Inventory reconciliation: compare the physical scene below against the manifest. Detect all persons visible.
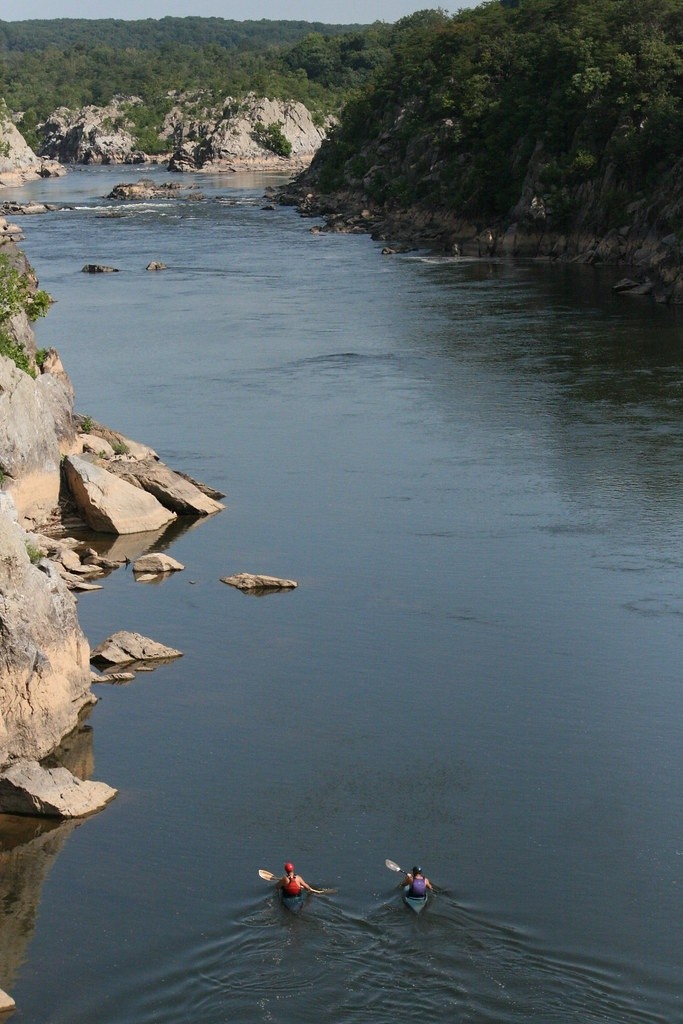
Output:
[401,865,433,898]
[275,863,319,898]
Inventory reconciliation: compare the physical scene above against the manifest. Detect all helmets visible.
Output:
[412,866,423,875]
[285,863,295,871]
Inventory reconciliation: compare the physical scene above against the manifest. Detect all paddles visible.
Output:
[385,858,439,893]
[258,869,338,894]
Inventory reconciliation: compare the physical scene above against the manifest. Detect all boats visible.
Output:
[282,897,304,918]
[403,875,428,914]
